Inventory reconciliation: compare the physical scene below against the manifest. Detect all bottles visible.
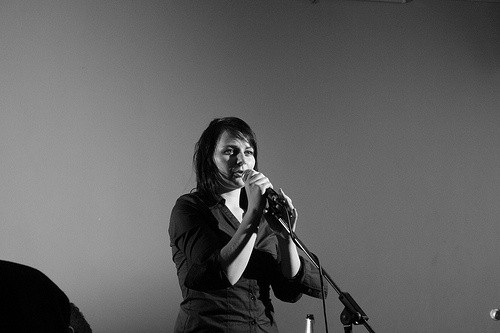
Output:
[304,314,315,333]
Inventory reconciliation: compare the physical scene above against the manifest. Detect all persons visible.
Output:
[168,116,327,333]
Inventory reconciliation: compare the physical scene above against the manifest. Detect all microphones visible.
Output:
[490,308,500,320]
[242,169,285,207]
